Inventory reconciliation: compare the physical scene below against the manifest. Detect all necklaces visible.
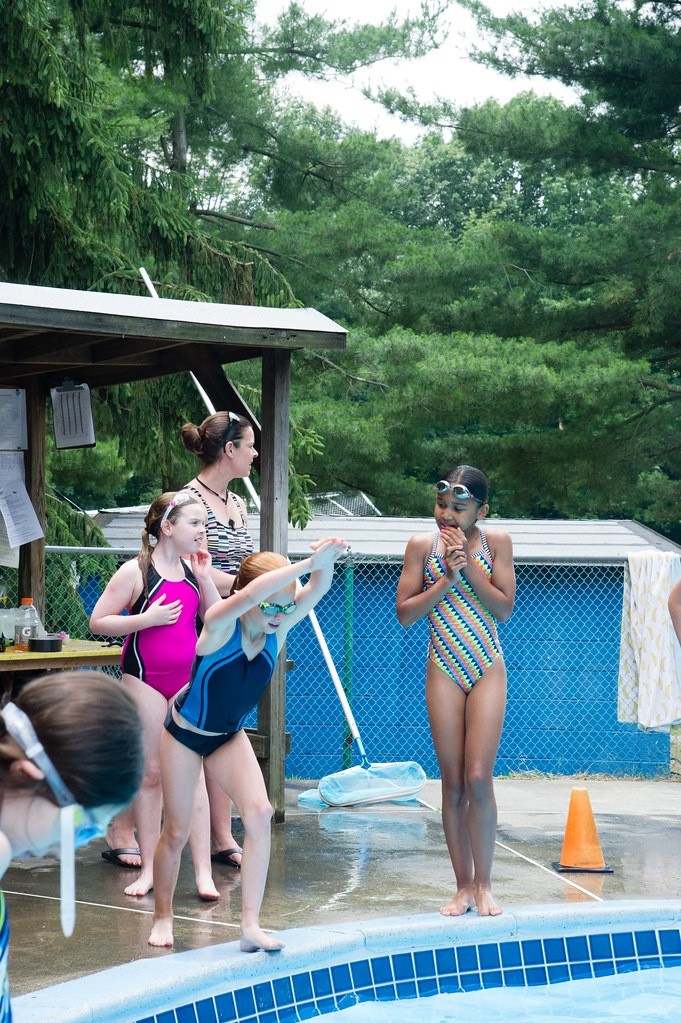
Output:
[195,475,235,532]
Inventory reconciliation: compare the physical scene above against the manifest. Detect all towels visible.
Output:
[617,549,681,733]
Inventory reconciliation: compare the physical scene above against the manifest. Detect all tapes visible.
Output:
[28,637,63,652]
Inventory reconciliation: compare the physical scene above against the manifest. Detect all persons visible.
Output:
[147,539,351,953]
[396,466,516,917]
[89,489,222,900]
[0,668,147,1022]
[102,410,259,870]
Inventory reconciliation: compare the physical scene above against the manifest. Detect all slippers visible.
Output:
[212,848,243,867]
[101,848,142,869]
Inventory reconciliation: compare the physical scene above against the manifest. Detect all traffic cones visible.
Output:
[550,788,614,873]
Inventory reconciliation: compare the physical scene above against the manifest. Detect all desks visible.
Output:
[0,638,123,699]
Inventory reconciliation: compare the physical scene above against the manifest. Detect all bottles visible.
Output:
[14,598,38,651]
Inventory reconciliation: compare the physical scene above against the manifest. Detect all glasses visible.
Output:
[222,411,240,453]
[435,480,485,506]
[160,488,201,529]
[233,589,297,617]
[33,760,104,851]
